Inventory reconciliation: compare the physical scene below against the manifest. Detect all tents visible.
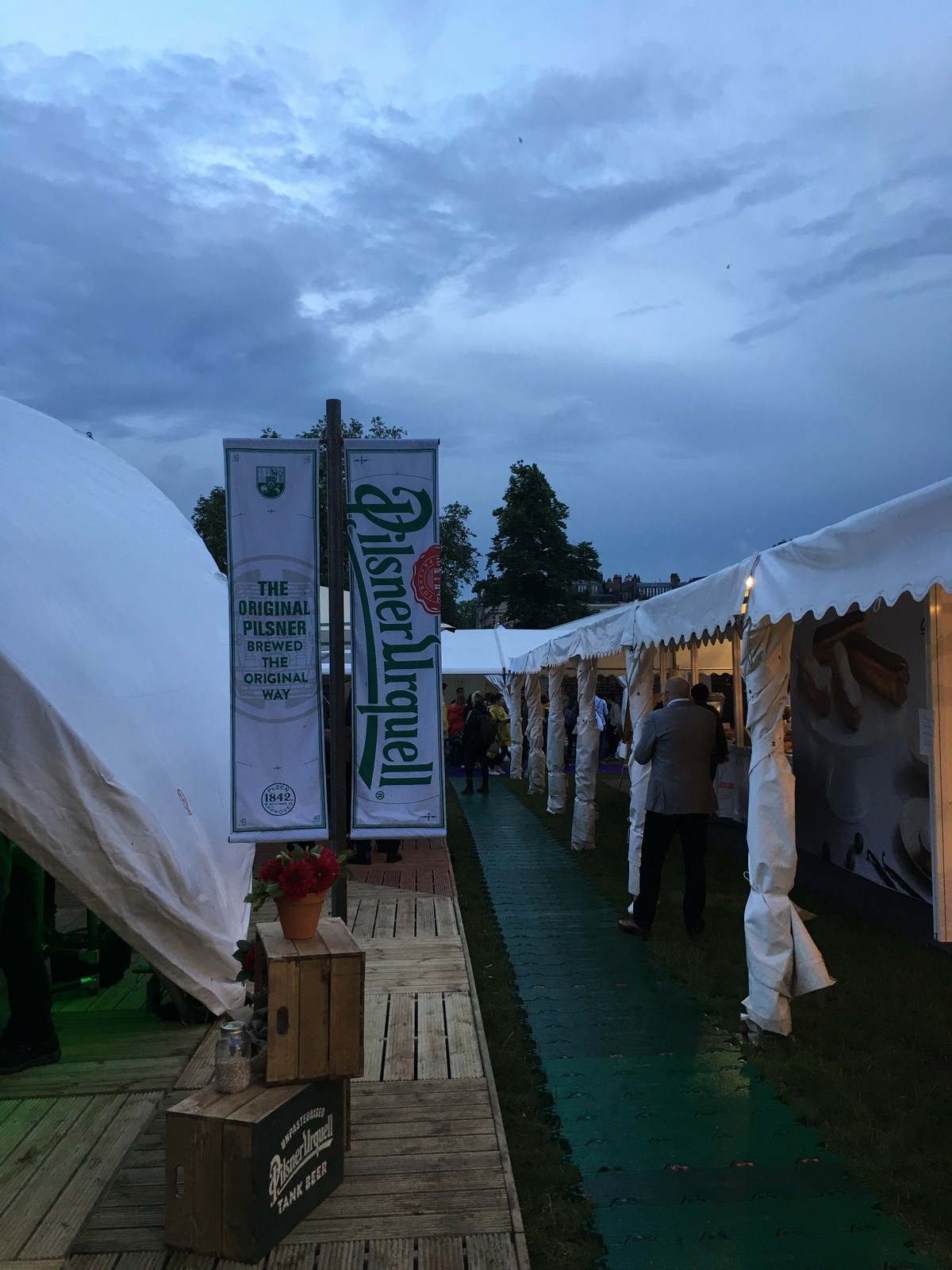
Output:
[504,478,952,1037]
[0,395,257,1017]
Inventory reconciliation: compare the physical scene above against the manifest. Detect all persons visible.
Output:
[690,683,729,784]
[541,694,549,754]
[594,695,609,765]
[344,687,403,865]
[720,675,747,727]
[0,833,61,1074]
[616,676,718,937]
[489,693,510,774]
[606,693,621,760]
[442,683,473,769]
[563,697,578,758]
[460,691,491,795]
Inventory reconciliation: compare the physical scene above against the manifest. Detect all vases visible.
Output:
[275,890,328,939]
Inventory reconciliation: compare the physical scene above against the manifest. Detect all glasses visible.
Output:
[659,690,669,700]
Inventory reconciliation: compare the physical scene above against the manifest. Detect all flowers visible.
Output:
[242,842,353,912]
[233,936,268,1014]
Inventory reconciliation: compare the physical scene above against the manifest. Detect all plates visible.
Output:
[900,797,932,882]
[800,660,907,745]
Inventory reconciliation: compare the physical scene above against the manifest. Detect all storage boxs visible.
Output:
[255,917,366,1086]
[163,1076,352,1265]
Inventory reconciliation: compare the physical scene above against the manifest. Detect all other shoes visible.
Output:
[690,920,707,937]
[461,787,474,795]
[488,767,501,775]
[494,764,506,774]
[477,786,489,794]
[619,920,650,939]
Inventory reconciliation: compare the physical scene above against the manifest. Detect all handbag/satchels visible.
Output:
[449,731,463,747]
[572,725,577,734]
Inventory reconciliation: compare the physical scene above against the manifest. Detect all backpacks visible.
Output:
[595,699,604,732]
[474,708,498,742]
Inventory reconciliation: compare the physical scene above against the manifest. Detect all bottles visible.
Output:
[214,1021,250,1093]
